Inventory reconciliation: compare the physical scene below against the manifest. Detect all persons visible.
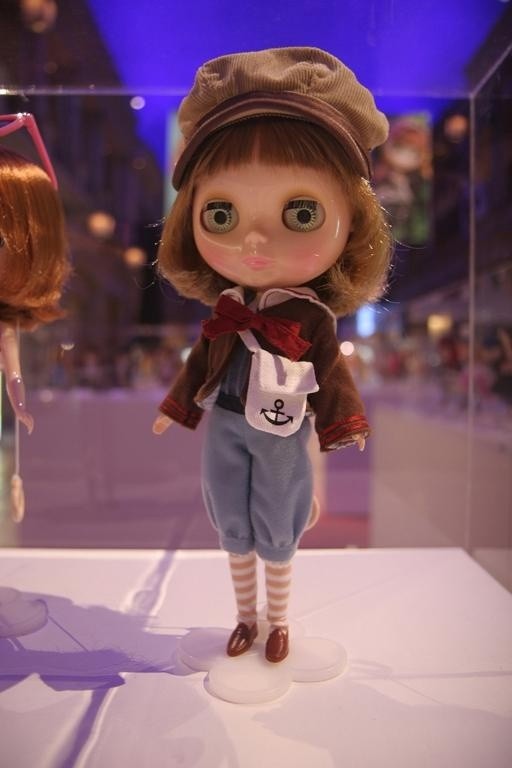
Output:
[151,47,391,664]
